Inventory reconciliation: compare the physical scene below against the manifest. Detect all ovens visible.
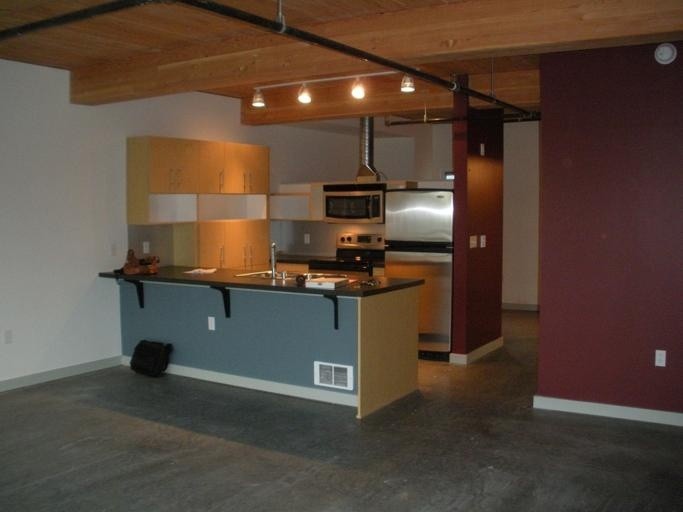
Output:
[310,267,370,277]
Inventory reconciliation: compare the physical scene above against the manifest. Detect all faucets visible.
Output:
[270,243,278,278]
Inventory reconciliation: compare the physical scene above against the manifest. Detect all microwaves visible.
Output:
[322,192,384,225]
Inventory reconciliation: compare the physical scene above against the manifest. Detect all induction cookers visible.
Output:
[310,250,386,266]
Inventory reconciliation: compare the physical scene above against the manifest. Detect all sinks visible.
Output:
[284,272,349,283]
[233,269,284,279]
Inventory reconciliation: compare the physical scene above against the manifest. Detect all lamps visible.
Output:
[249,69,416,109]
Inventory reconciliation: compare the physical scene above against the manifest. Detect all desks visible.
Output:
[96,266,426,420]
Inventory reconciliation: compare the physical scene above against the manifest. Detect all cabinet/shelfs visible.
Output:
[124,136,198,194]
[125,195,268,224]
[223,221,272,269]
[173,222,223,266]
[198,139,223,194]
[223,141,268,194]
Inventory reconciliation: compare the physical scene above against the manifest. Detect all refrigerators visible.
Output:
[382,190,454,357]
